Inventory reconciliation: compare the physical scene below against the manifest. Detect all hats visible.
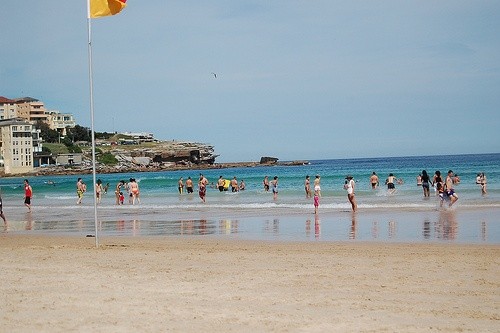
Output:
[345,175,353,179]
[97,178,102,183]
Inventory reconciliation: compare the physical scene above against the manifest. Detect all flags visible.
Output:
[87,0,127,20]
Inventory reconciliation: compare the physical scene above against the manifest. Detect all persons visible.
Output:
[385,171,406,193]
[304,173,322,214]
[369,171,379,190]
[216,175,245,192]
[96,178,109,203]
[416,170,461,208]
[0,192,6,224]
[76,177,87,205]
[197,174,209,205]
[476,172,488,195]
[343,174,359,213]
[264,175,280,200]
[115,178,142,205]
[178,176,194,194]
[23,179,33,213]
